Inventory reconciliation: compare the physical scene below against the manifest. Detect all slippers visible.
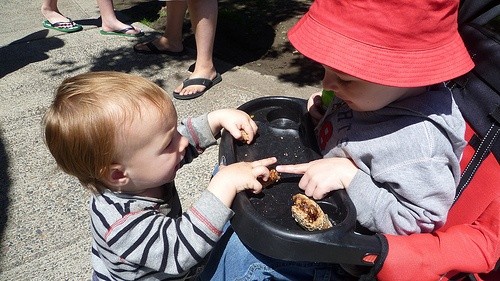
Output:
[100,24,145,37]
[172,72,222,100]
[134,38,184,56]
[43,16,82,33]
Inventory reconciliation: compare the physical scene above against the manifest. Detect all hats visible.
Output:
[286,0,476,88]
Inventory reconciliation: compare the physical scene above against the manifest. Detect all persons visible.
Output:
[132,0,222,100]
[40,0,145,36]
[41,71,277,281]
[204,0,475,281]
